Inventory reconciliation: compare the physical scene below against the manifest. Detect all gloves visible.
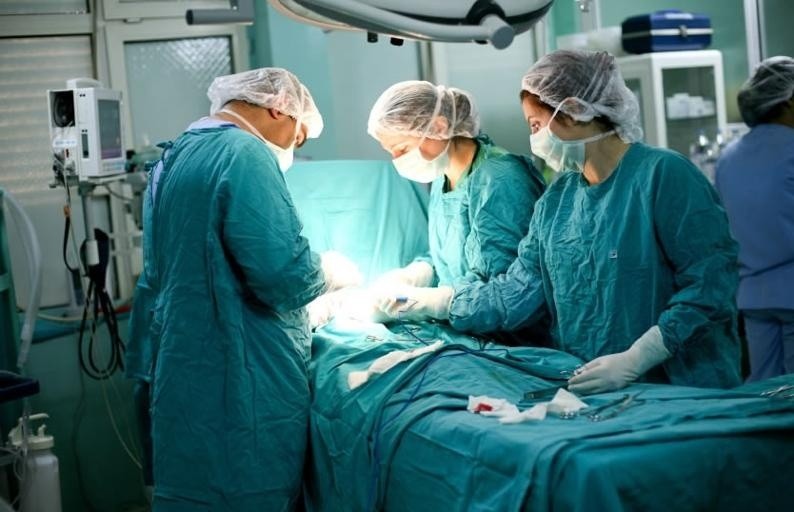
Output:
[321,251,362,291]
[566,322,672,398]
[374,287,455,322]
[384,262,435,287]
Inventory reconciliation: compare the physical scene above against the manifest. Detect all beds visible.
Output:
[246,160,794,511]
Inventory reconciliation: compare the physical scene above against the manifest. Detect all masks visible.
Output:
[529,97,596,176]
[392,117,456,184]
[259,108,301,176]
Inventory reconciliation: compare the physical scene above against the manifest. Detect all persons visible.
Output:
[353,77,553,346]
[371,46,745,405]
[700,56,794,379]
[122,65,335,509]
